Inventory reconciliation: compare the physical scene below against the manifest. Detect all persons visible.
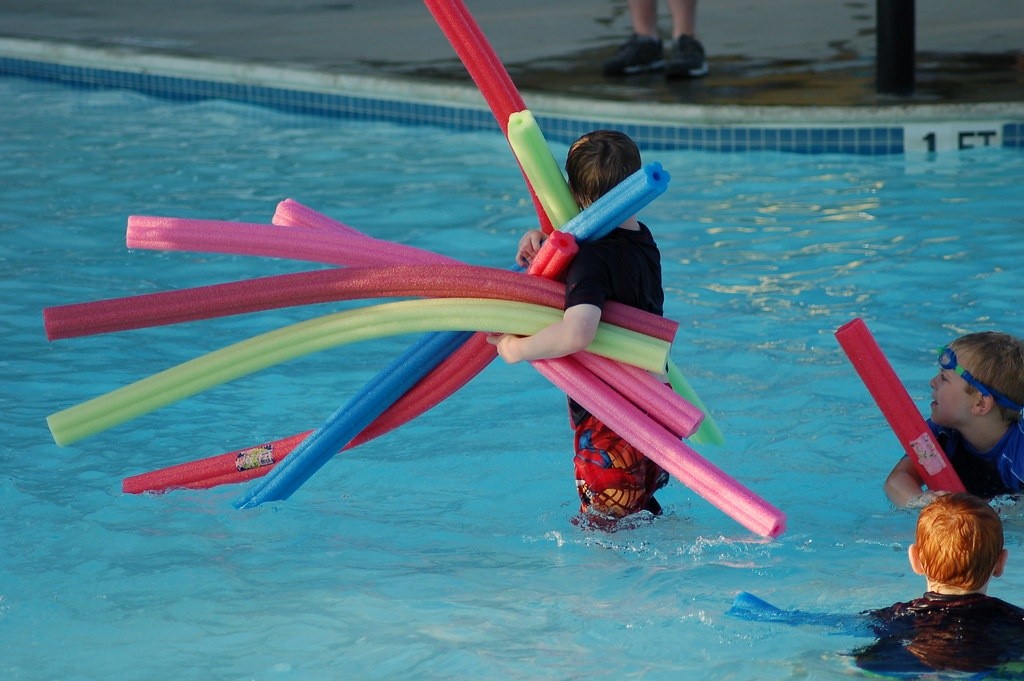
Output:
[598,0,709,79]
[855,490,1024,681]
[883,331,1024,512]
[486,128,681,535]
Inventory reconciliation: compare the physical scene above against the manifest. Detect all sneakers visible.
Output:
[665,33,708,78]
[602,33,665,75]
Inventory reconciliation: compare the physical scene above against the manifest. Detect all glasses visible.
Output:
[939,341,1024,412]
